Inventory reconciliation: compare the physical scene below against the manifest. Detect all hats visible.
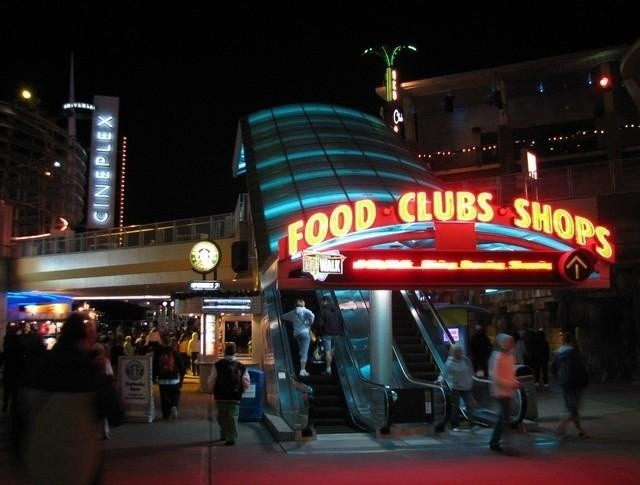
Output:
[222,341,238,353]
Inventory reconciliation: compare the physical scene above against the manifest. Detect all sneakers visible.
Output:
[489,441,506,452]
[298,367,312,376]
[226,440,236,446]
[169,406,177,422]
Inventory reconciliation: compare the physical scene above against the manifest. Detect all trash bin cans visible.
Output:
[239,368,264,424]
[195,357,222,393]
[512,364,539,419]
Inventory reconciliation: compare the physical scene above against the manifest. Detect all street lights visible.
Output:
[360,40,420,134]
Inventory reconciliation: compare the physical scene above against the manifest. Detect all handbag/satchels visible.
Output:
[312,345,326,361]
[309,329,317,343]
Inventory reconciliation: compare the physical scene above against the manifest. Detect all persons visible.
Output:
[550,332,594,440]
[313,295,343,377]
[469,315,552,388]
[1,306,253,484]
[485,333,528,453]
[432,344,481,434]
[281,298,317,377]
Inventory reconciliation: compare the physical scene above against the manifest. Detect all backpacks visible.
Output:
[158,349,178,374]
